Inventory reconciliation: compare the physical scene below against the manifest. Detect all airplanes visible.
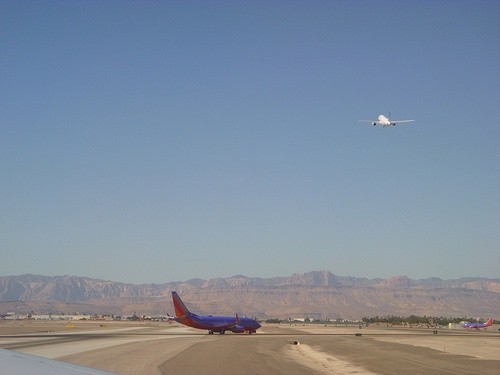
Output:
[168,292,261,336]
[464,316,494,331]
[360,113,416,127]
